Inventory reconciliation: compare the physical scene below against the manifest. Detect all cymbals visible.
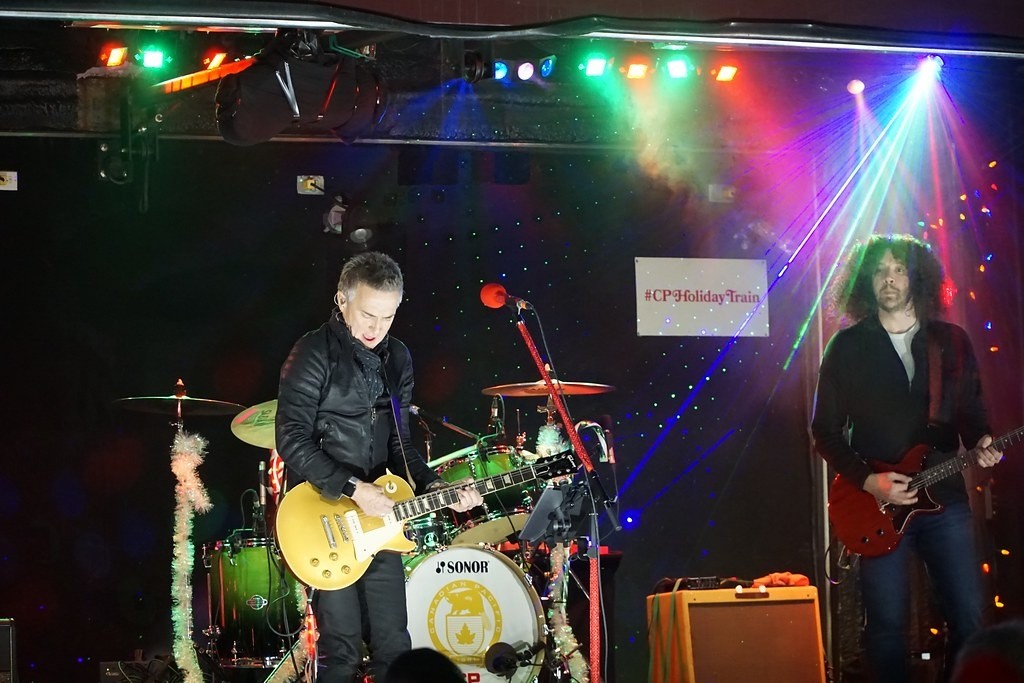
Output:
[480,362,615,397]
[230,398,278,450]
[114,376,248,417]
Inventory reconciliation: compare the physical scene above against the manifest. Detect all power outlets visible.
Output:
[0,171,18,191]
[708,183,738,203]
[297,175,325,195]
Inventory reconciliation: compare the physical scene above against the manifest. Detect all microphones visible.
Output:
[647,585,827,683]
[487,398,498,435]
[480,283,532,311]
[537,364,551,414]
[494,657,517,673]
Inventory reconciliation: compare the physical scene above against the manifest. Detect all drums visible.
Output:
[433,443,534,549]
[360,542,549,683]
[206,537,301,669]
[403,516,446,556]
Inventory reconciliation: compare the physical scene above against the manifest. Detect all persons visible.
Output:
[384,647,468,683]
[809,234,1003,683]
[274,252,483,683]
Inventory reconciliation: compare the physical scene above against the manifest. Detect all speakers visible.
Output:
[0,625,15,683]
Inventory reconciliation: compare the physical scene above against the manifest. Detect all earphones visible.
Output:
[340,298,345,306]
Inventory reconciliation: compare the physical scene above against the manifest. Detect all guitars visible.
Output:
[827,424,1024,559]
[271,448,579,593]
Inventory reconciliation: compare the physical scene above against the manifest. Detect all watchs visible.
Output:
[342,476,359,498]
